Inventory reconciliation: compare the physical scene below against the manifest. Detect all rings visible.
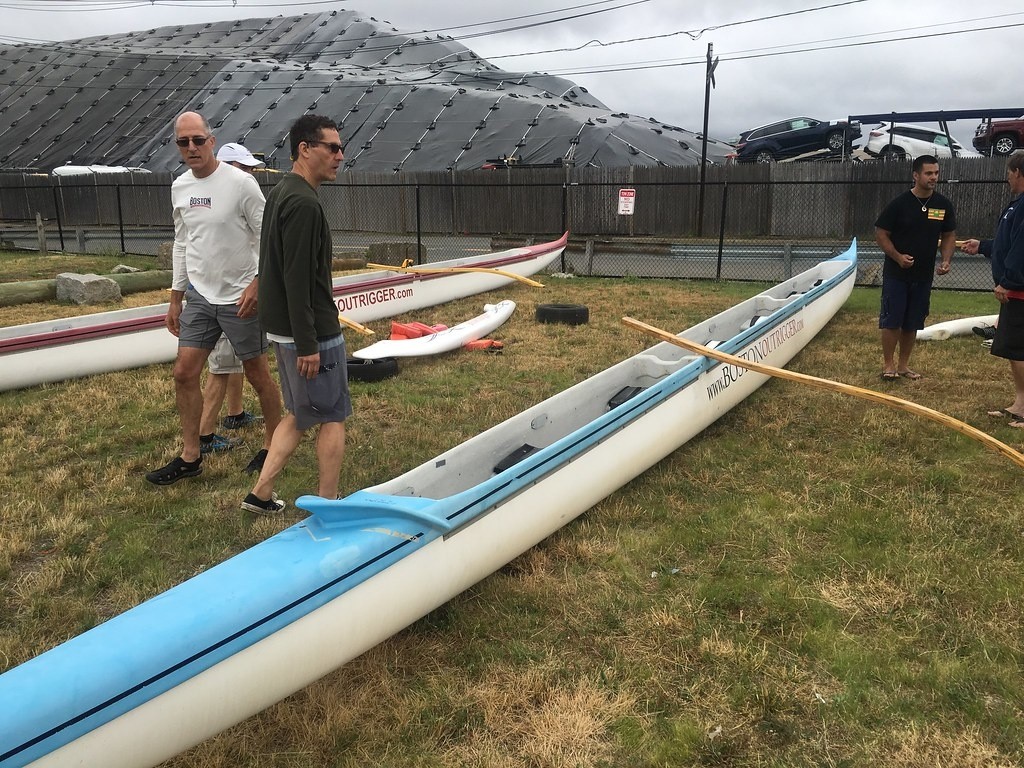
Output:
[252,307,256,311]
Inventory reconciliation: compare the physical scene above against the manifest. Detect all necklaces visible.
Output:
[912,189,932,212]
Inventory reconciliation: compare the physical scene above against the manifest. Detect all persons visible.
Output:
[874,155,958,380]
[241,115,353,515]
[144,110,283,484]
[961,148,1024,428]
[199,142,267,453]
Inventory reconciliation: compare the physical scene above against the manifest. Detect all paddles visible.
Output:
[365,262,546,289]
[165,287,376,336]
[621,315,1024,471]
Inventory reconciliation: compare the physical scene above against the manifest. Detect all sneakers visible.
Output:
[336,493,343,500]
[240,492,285,516]
[972,323,997,339]
[221,412,265,430]
[199,435,244,454]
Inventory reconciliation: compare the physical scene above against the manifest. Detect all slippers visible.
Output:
[897,370,923,380]
[880,371,901,381]
[1008,417,1024,429]
[987,409,1022,419]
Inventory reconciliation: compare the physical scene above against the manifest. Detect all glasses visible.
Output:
[175,134,211,148]
[298,139,345,155]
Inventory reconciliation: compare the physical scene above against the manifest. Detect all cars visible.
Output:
[863,121,985,169]
[972,114,1024,157]
[733,116,862,166]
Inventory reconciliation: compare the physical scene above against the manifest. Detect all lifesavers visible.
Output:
[347,356,400,384]
[536,303,591,327]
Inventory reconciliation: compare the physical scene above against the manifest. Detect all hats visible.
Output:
[216,143,266,169]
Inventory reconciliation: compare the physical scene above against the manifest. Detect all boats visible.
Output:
[352,298,516,360]
[0,229,569,396]
[1,236,859,768]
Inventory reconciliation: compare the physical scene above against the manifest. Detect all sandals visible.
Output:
[146,454,203,485]
[241,448,268,477]
